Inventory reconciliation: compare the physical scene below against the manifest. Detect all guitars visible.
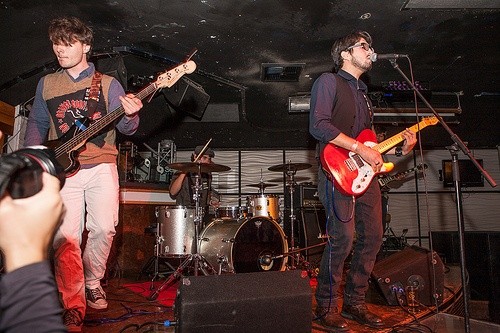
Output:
[38,47,199,178]
[320,116,442,197]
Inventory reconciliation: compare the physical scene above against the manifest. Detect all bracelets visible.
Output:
[395,148,402,157]
[352,141,358,151]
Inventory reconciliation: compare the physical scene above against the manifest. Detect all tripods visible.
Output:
[134,167,217,302]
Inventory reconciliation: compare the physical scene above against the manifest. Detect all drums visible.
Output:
[154,193,288,273]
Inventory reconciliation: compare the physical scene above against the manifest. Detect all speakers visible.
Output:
[367,243,445,307]
[282,208,327,271]
[172,270,313,333]
[161,76,210,120]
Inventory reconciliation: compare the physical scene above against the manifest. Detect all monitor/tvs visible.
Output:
[442,159,484,187]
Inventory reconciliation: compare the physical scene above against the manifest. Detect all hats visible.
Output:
[195,146,214,158]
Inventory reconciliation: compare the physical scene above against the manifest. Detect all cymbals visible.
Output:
[268,163,313,172]
[246,184,278,188]
[168,162,231,175]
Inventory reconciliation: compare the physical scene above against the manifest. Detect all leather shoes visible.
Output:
[340,303,385,329]
[316,305,351,330]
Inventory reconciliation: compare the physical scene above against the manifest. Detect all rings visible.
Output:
[376,162,379,165]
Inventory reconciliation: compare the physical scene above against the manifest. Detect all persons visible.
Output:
[169,146,222,213]
[0,172,69,333]
[309,31,417,330]
[23,16,143,331]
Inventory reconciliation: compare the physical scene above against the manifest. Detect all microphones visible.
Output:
[370,52,408,63]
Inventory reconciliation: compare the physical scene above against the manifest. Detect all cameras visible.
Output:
[0,143,66,204]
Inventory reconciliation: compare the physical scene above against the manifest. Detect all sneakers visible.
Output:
[62,308,84,332]
[85,286,108,309]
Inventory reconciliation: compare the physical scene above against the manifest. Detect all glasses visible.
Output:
[345,41,374,53]
